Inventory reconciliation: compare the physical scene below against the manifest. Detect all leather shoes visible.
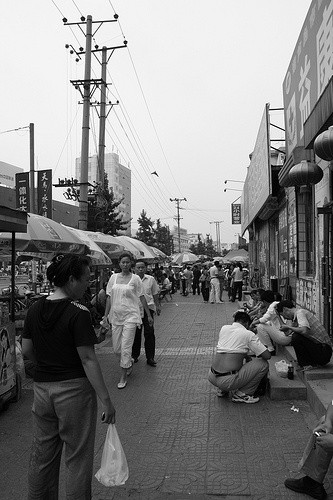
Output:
[132,357,138,363]
[147,357,158,364]
[285,476,328,500]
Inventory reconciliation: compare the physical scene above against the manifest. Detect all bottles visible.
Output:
[288,360,294,380]
[100,321,110,329]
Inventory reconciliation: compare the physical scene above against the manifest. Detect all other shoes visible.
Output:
[181,293,242,304]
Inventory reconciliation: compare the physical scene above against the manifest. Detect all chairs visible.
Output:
[157,282,173,302]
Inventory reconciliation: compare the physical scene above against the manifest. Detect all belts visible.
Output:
[211,366,237,377]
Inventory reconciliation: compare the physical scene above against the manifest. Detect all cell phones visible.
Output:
[102,412,105,421]
[315,432,320,438]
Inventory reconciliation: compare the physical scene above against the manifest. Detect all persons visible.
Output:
[159,261,244,304]
[101,253,154,388]
[243,288,282,325]
[250,290,295,356]
[130,259,161,366]
[78,279,108,329]
[208,312,271,404]
[275,301,333,372]
[22,253,117,500]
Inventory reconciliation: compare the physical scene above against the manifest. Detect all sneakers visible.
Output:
[296,365,313,372]
[117,380,128,389]
[232,392,260,404]
[217,389,229,397]
[126,358,134,376]
